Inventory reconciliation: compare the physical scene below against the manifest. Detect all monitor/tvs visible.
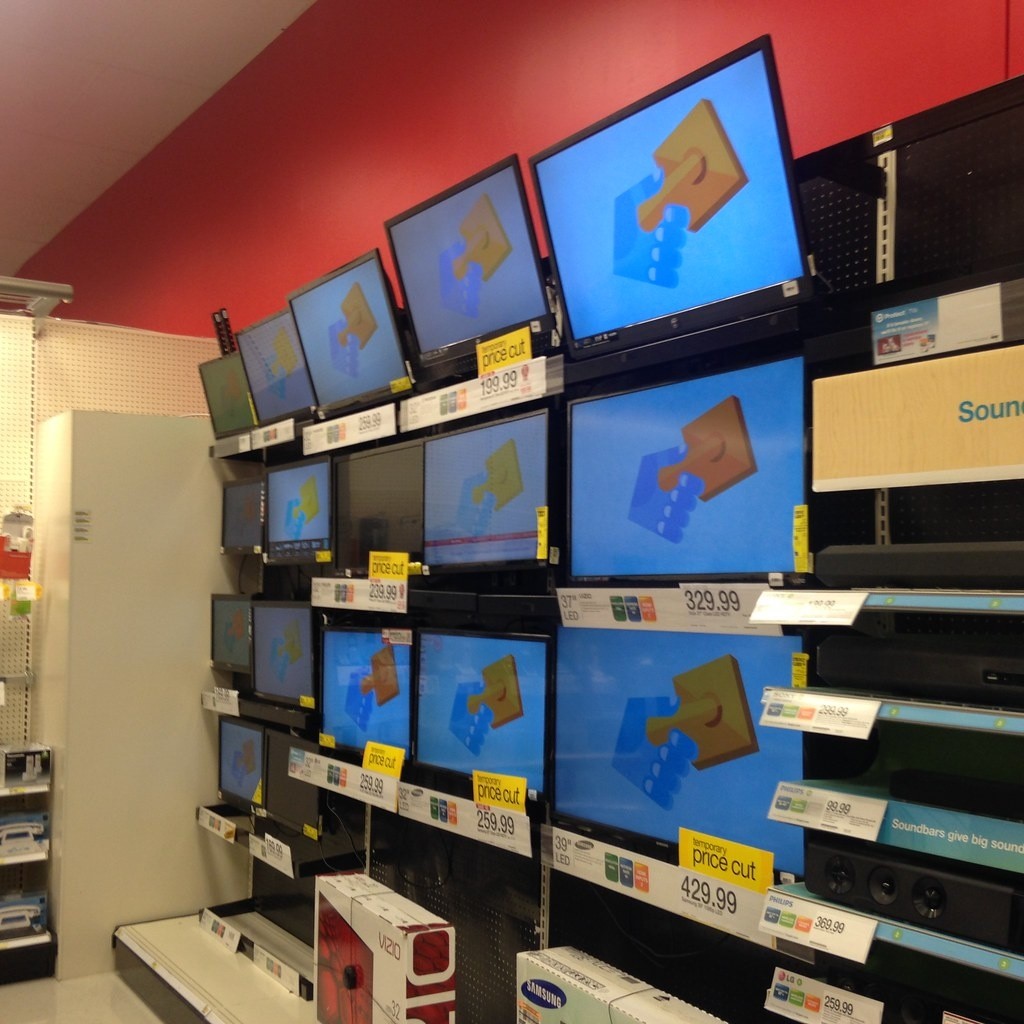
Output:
[200,36,817,891]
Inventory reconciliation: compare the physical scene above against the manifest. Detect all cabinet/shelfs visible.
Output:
[194,306,1024,1024]
[0,744,58,985]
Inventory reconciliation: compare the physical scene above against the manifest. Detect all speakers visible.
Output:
[803,837,1024,956]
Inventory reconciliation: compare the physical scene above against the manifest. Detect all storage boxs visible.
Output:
[513,948,730,1024]
[314,873,458,1024]
